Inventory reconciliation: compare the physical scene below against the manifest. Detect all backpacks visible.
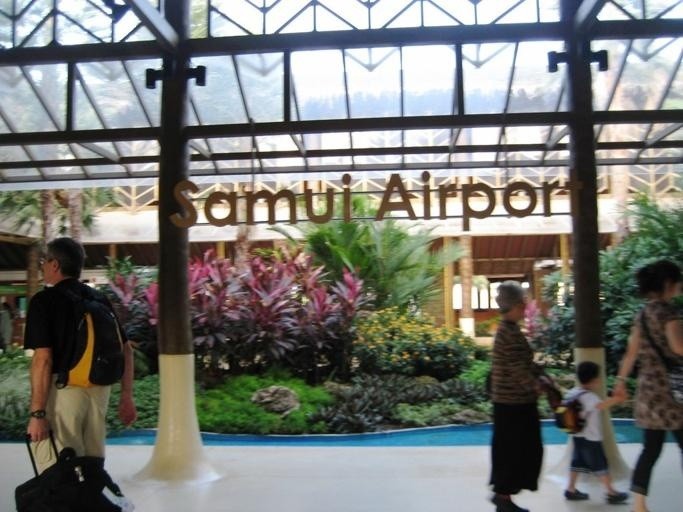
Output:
[46,286,124,390]
[554,391,592,436]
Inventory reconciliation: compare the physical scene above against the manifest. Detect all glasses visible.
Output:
[39,256,49,264]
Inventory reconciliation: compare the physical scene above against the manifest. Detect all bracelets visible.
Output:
[29,409,48,420]
[615,376,627,383]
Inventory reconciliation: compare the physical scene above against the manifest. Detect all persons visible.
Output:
[562,361,629,505]
[485,281,557,512]
[610,258,682,512]
[23,237,138,476]
[0,302,16,354]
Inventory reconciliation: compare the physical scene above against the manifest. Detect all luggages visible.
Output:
[15,429,124,512]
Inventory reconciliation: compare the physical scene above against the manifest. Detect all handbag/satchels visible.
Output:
[486,371,490,394]
[663,358,682,374]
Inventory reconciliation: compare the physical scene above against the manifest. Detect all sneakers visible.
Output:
[564,489,589,500]
[607,492,628,503]
[492,494,529,512]
